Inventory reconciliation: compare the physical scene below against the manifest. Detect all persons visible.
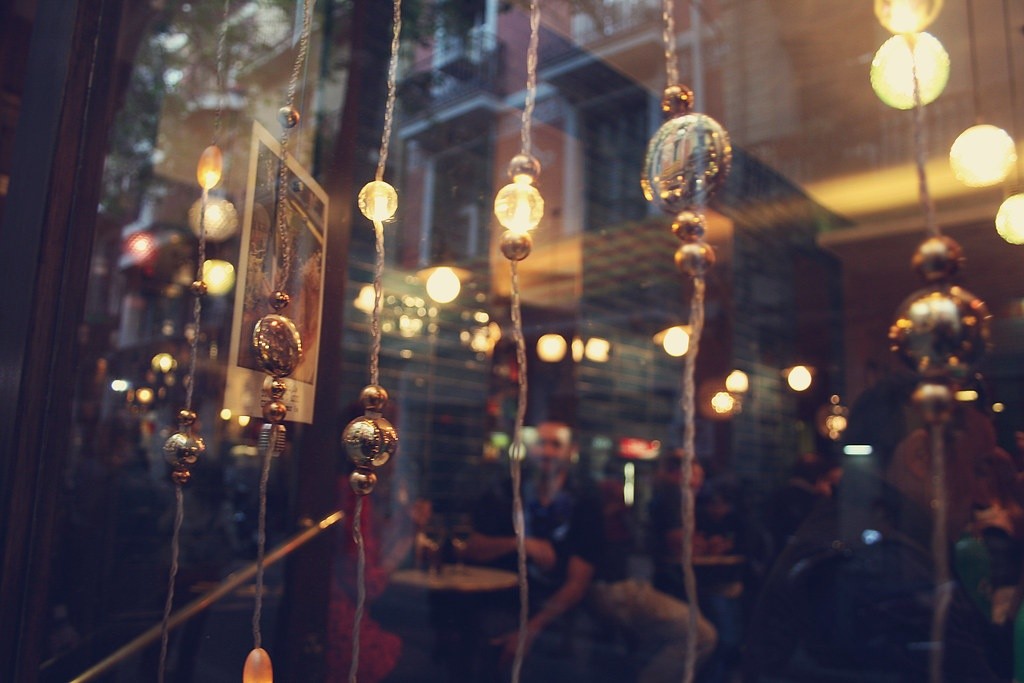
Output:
[585,376,1022,683]
[446,416,602,676]
[327,397,433,683]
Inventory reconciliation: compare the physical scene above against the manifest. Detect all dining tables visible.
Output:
[390,560,524,682]
